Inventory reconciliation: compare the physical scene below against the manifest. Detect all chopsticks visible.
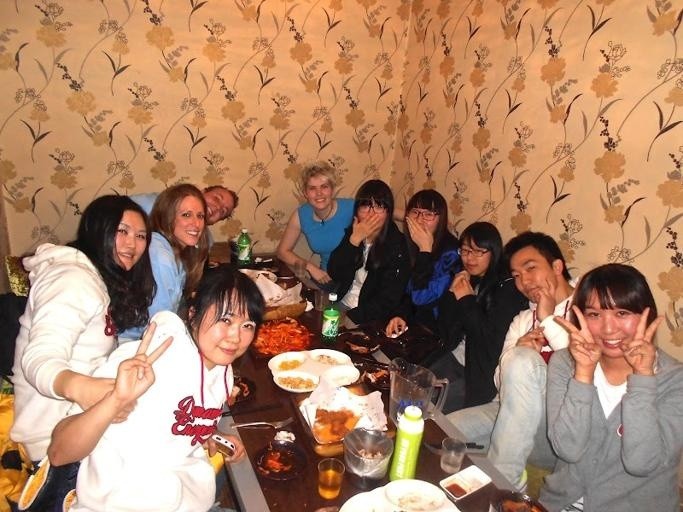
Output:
[420,442,485,450]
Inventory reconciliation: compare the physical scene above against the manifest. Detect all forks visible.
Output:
[229,417,294,430]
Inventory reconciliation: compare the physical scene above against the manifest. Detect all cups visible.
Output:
[317,457,346,500]
[343,429,394,491]
[388,356,450,429]
[313,290,329,312]
[338,306,346,327]
[439,437,467,474]
[294,260,306,281]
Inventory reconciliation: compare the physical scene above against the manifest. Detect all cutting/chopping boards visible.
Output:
[287,374,398,457]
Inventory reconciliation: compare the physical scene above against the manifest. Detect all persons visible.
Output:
[48,264,265,511]
[130,184,238,252]
[444,230,577,495]
[274,165,355,285]
[117,184,207,347]
[359,189,463,359]
[537,263,683,511]
[322,180,402,328]
[10,194,157,470]
[412,222,503,409]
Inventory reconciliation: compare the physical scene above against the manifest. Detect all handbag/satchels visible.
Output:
[357,313,444,364]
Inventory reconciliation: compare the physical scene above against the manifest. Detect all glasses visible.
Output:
[456,248,490,257]
[358,202,387,213]
[407,208,439,221]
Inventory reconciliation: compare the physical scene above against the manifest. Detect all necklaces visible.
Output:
[314,200,334,225]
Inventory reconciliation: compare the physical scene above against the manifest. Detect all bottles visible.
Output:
[320,292,341,346]
[387,404,425,481]
[237,228,252,269]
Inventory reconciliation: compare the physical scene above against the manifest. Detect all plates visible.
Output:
[231,374,258,404]
[344,333,382,355]
[490,490,550,512]
[253,440,308,482]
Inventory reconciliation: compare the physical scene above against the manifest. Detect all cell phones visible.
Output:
[212,435,235,457]
